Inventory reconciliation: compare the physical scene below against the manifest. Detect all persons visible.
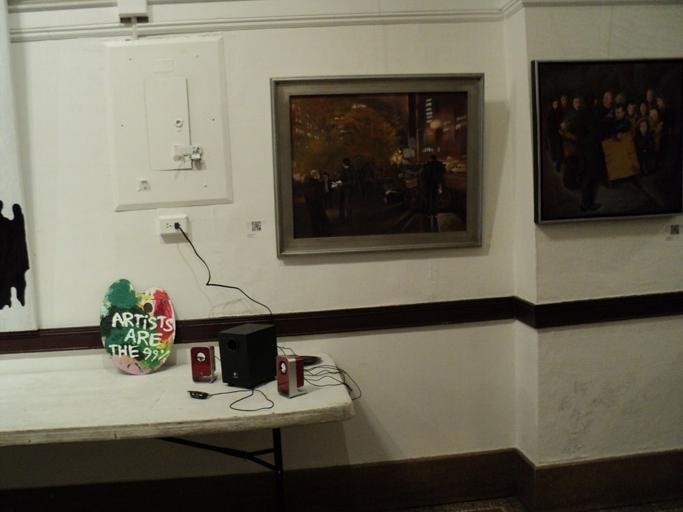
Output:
[292,145,448,233]
[544,85,677,213]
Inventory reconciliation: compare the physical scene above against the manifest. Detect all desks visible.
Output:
[0,348,356,512]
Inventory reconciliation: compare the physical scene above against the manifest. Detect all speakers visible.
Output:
[191,347,216,384]
[219,326,276,388]
[277,353,305,398]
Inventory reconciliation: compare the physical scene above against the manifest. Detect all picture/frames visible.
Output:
[268,68,489,259]
[528,57,683,226]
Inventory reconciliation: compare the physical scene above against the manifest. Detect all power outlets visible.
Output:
[158,214,190,238]
